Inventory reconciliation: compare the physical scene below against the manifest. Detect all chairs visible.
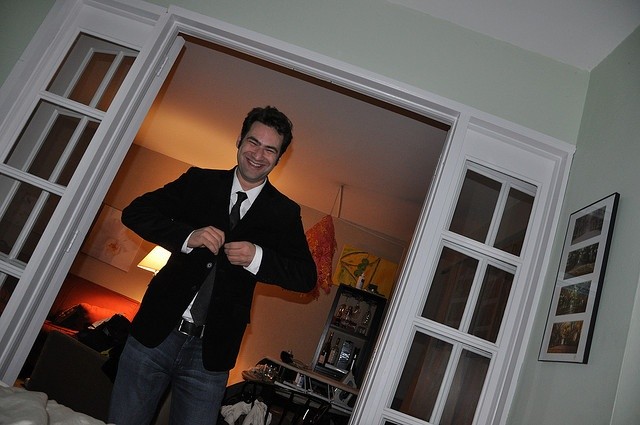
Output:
[263,388,332,425]
[239,381,294,425]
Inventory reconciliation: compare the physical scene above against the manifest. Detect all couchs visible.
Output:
[42,279,141,343]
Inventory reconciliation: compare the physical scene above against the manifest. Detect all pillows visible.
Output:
[54,302,113,327]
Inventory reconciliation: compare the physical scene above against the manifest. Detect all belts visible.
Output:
[176,319,205,340]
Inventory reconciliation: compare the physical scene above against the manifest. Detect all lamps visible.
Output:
[137,245,171,275]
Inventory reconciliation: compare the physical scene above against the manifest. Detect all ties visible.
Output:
[190,191,248,327]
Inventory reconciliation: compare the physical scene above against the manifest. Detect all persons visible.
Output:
[107,105,317,424]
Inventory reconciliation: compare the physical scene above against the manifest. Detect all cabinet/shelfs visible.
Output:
[309,283,388,389]
[258,355,360,422]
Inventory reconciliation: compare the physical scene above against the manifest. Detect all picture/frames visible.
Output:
[538,190,621,366]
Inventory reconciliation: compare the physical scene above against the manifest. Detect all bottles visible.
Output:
[355,272,365,290]
[296,374,305,389]
[327,338,342,365]
[316,331,335,367]
